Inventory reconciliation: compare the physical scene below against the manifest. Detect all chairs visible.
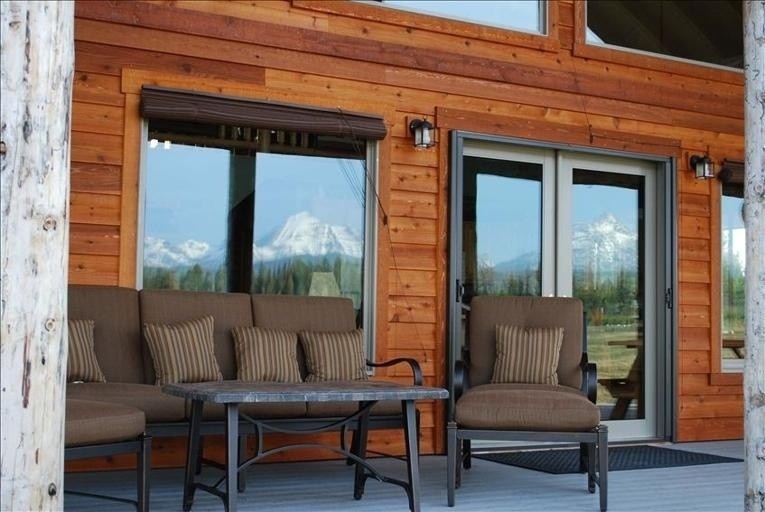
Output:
[445,293,609,511]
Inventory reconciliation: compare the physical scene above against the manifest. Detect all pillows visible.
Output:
[489,323,565,387]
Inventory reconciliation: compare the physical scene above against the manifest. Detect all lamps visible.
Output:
[690,153,715,180]
[410,118,437,149]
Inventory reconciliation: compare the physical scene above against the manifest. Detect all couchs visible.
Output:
[68,283,422,495]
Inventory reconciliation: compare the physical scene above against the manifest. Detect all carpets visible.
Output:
[469,445,747,475]
